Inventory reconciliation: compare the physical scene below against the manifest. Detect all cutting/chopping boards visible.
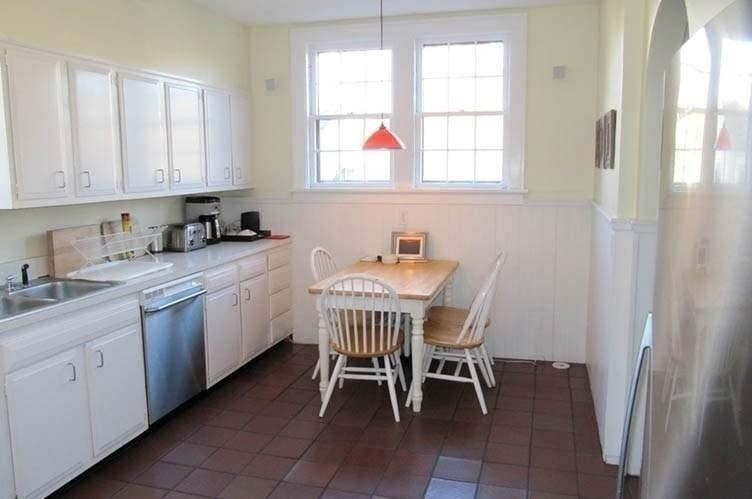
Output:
[48,218,146,279]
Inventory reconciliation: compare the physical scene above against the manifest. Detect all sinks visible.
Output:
[1,290,57,320]
[8,280,114,302]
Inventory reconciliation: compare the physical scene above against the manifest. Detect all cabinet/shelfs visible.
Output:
[202,251,276,393]
[0,291,151,498]
[264,238,295,349]
[114,64,208,202]
[2,44,123,213]
[197,79,256,195]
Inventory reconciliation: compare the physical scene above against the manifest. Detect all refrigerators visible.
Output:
[611,0,752,498]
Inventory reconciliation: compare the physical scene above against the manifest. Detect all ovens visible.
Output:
[140,271,206,426]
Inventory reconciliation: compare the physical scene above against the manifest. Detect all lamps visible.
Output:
[357,1,406,152]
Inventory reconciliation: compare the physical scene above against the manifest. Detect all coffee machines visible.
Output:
[185,195,221,244]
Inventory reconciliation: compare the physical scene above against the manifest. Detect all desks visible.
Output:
[308,258,464,415]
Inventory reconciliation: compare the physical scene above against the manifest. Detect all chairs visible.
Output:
[406,248,513,415]
[307,245,385,388]
[317,271,408,426]
[662,237,752,453]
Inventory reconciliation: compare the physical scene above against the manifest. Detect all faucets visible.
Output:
[21,263,29,287]
[5,273,18,291]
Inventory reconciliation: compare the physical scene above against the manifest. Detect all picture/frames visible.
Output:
[391,230,428,261]
[593,110,618,171]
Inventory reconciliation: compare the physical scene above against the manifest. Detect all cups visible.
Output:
[148,225,163,253]
[218,219,241,234]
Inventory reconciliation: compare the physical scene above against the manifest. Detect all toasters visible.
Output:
[166,222,206,252]
